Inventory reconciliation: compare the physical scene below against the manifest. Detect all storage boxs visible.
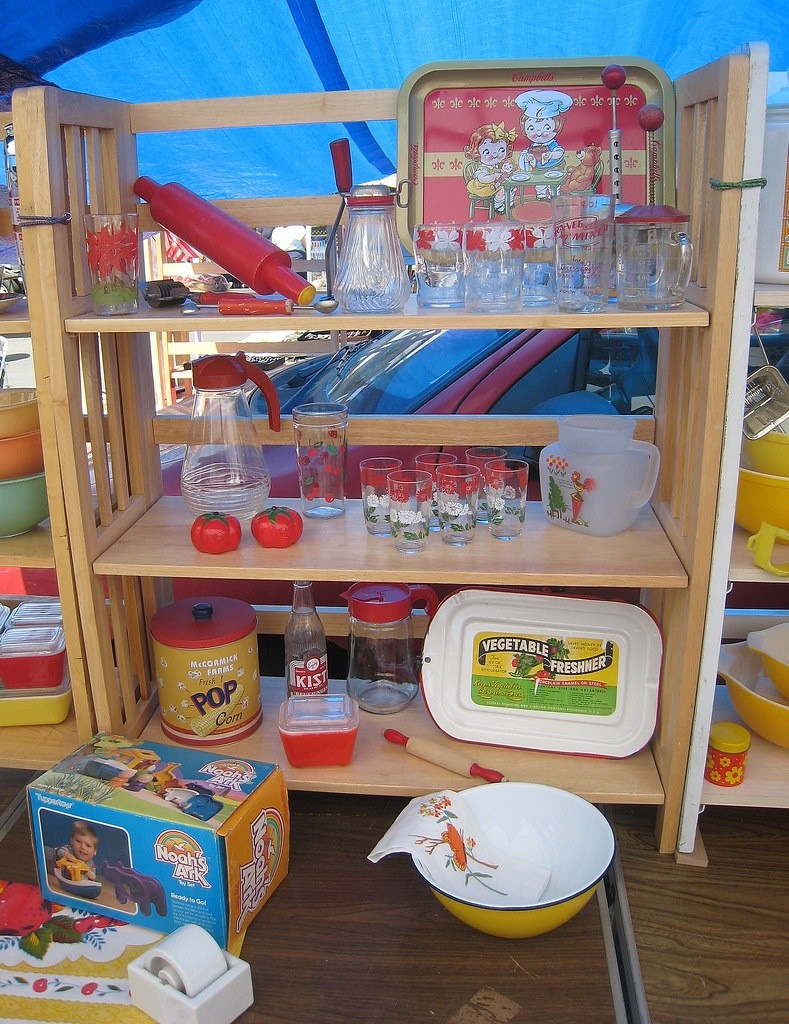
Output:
[278,694,361,767]
[0,601,71,728]
[26,732,290,950]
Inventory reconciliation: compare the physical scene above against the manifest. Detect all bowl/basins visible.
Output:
[717,622,789,748]
[412,782,614,939]
[279,695,359,767]
[0,599,73,726]
[0,388,51,538]
[735,416,789,544]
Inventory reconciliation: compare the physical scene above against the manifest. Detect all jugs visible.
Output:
[339,582,438,715]
[180,350,280,521]
[333,184,410,312]
[538,414,661,537]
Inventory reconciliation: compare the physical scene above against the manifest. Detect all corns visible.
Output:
[190,684,244,737]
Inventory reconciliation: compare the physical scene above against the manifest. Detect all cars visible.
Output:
[91,308,789,680]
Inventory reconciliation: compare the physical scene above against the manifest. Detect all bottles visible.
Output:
[285,581,327,695]
[755,106,789,285]
[4,122,47,297]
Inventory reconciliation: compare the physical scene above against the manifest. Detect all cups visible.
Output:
[82,214,142,315]
[612,202,693,311]
[518,218,553,306]
[292,403,348,520]
[413,224,466,307]
[463,221,526,308]
[550,195,612,313]
[415,453,457,531]
[386,469,432,553]
[435,464,480,546]
[465,447,507,523]
[484,458,529,540]
[359,457,403,536]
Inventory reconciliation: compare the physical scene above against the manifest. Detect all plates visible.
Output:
[420,586,663,759]
[0,293,24,312]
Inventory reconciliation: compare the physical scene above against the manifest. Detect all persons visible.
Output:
[271,225,308,279]
[47,821,99,880]
[44,846,75,863]
[256,227,274,241]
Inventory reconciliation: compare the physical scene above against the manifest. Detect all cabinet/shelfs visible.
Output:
[3,40,789,864]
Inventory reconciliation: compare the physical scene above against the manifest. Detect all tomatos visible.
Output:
[190,511,242,554]
[251,505,303,548]
